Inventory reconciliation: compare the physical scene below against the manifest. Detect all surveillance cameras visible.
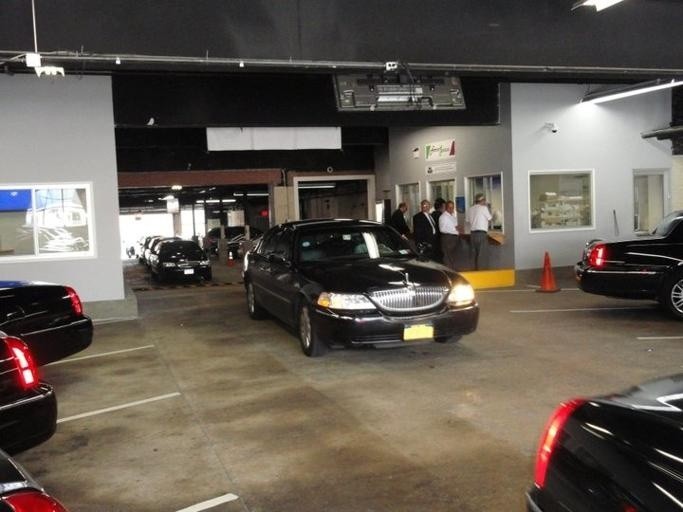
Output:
[552,125,558,133]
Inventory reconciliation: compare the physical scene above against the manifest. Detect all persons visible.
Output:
[430,196,446,244]
[390,201,410,239]
[412,199,436,259]
[464,192,498,268]
[437,200,463,268]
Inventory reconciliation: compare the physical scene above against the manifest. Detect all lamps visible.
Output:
[580,77,682,105]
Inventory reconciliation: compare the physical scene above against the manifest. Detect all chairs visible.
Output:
[299,237,327,260]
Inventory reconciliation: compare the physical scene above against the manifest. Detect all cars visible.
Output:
[0,278,94,371]
[0,446,69,511]
[123,223,264,286]
[517,370,683,512]
[238,216,481,359]
[573,211,683,322]
[0,331,58,456]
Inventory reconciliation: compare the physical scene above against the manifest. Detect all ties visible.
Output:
[429,215,434,226]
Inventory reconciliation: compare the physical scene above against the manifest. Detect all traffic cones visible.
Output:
[532,250,564,293]
